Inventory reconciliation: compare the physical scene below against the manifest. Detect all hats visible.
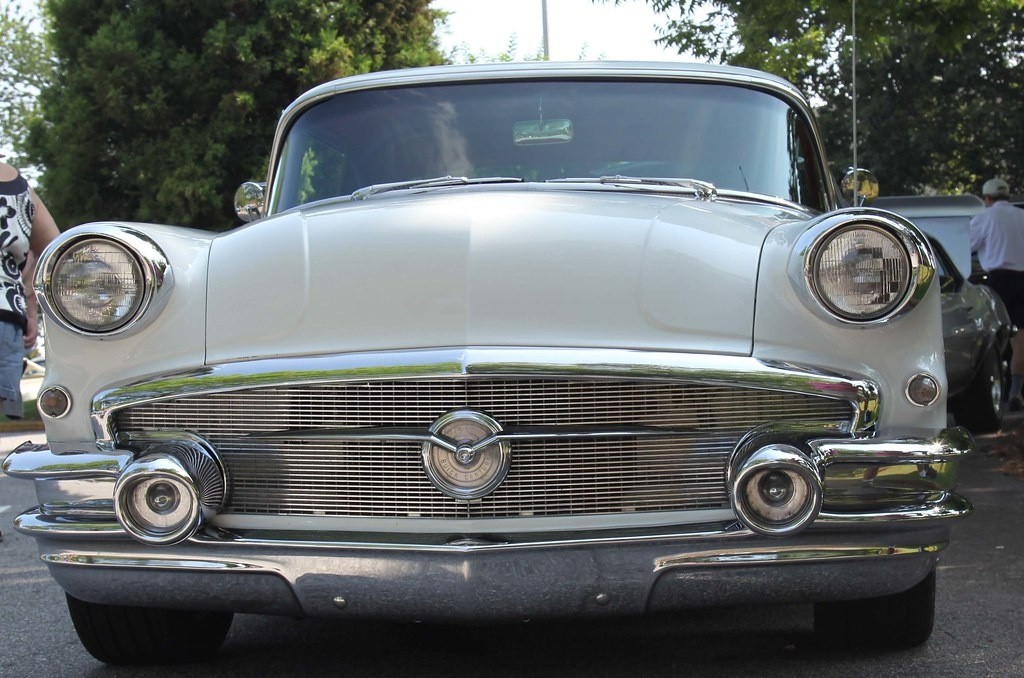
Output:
[982,179,1009,193]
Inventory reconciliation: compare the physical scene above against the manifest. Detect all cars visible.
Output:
[0,62,974,669]
[923,229,1018,431]
[25,355,46,374]
[862,194,987,283]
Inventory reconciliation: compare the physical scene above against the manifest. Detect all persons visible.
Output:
[0,162,60,542]
[969,180,1024,410]
